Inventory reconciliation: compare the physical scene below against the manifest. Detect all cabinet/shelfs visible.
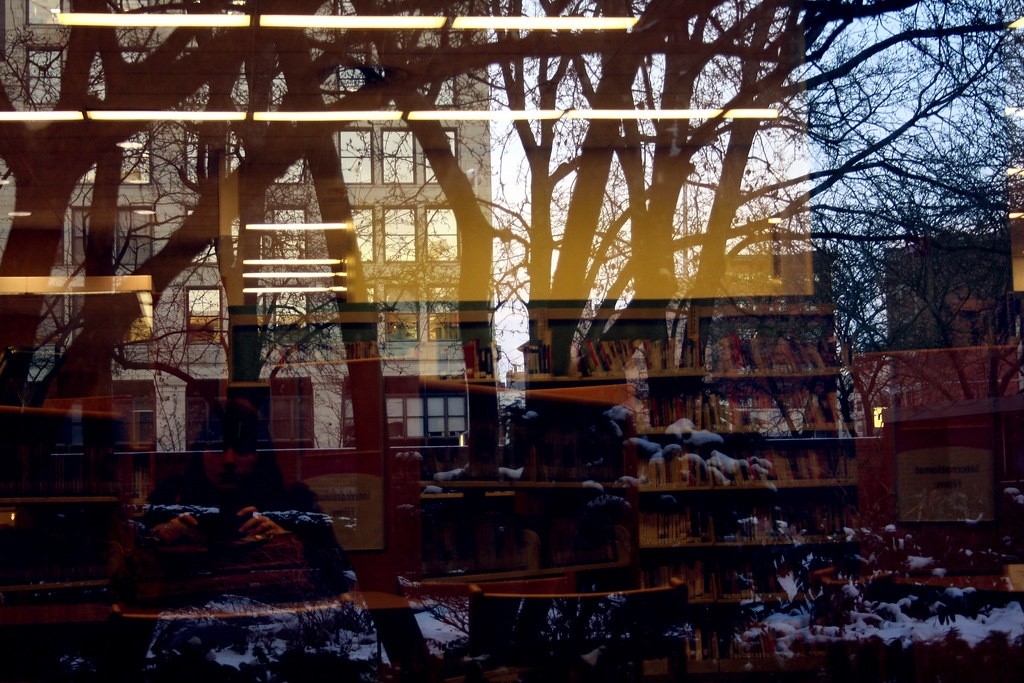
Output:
[422,303,876,677]
[0,405,141,606]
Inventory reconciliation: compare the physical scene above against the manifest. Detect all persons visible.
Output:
[131,398,356,611]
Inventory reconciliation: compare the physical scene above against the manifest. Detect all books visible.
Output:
[258,336,384,367]
[453,335,867,661]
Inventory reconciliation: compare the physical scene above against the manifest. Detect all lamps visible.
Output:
[315,63,412,89]
[195,148,218,177]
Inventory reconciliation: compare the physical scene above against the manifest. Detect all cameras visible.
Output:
[190,511,253,540]
